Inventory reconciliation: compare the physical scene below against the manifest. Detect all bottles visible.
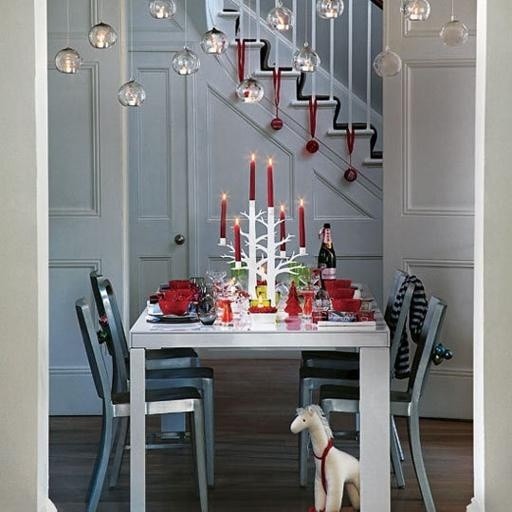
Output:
[222,301,235,327]
[301,291,315,315]
[318,223,337,289]
[200,301,216,326]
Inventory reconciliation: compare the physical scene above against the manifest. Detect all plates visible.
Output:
[156,312,197,322]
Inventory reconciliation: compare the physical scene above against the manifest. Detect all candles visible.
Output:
[279,197,305,251]
[249,152,274,207]
[233,217,241,261]
[220,192,228,237]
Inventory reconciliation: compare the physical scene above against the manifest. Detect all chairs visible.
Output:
[298,269,448,512]
[74,269,217,512]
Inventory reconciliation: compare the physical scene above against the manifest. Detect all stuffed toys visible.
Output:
[290,405,360,512]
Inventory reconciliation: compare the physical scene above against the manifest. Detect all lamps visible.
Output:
[88,1,119,51]
[148,0,178,21]
[115,80,147,107]
[265,1,296,33]
[53,1,85,75]
[291,1,324,74]
[438,1,469,50]
[370,1,404,78]
[397,0,435,23]
[234,74,265,105]
[199,26,231,57]
[170,47,202,77]
[314,0,347,21]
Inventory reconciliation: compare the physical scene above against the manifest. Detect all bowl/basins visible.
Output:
[170,281,191,287]
[325,280,351,292]
[159,299,186,316]
[331,286,357,300]
[164,287,191,301]
[333,299,361,313]
